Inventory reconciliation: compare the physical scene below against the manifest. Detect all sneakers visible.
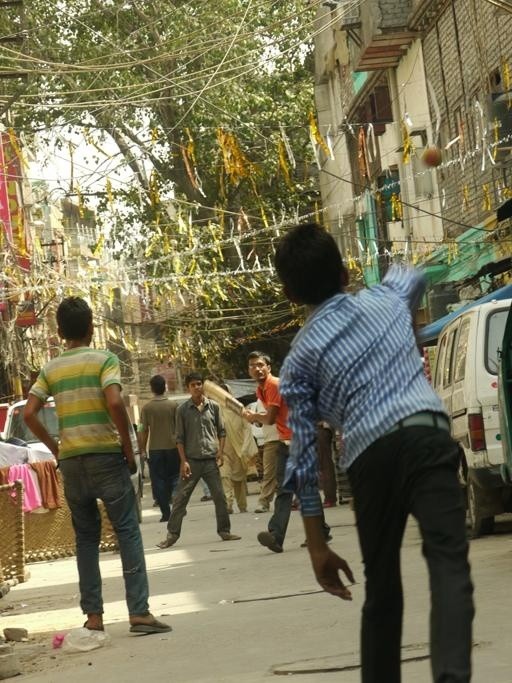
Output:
[258,532,283,553]
[301,524,330,547]
[255,505,267,513]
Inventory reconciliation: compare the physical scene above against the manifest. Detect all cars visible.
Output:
[159,379,291,477]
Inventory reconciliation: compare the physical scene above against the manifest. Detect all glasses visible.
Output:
[247,362,267,368]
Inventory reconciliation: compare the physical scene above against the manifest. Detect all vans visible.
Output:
[433,297,512,536]
[0,394,143,520]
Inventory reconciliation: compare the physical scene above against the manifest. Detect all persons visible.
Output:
[252,394,297,515]
[22,294,174,637]
[202,378,259,515]
[238,349,332,554]
[271,221,475,683]
[153,370,242,551]
[134,373,183,524]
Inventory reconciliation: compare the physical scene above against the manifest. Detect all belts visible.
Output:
[380,412,450,434]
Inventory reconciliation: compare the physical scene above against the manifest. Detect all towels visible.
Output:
[8,463,44,516]
[33,461,65,513]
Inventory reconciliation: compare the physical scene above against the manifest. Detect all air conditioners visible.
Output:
[335,1,365,32]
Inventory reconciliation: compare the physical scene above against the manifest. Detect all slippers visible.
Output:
[130,621,170,633]
[82,621,104,631]
[201,496,211,501]
